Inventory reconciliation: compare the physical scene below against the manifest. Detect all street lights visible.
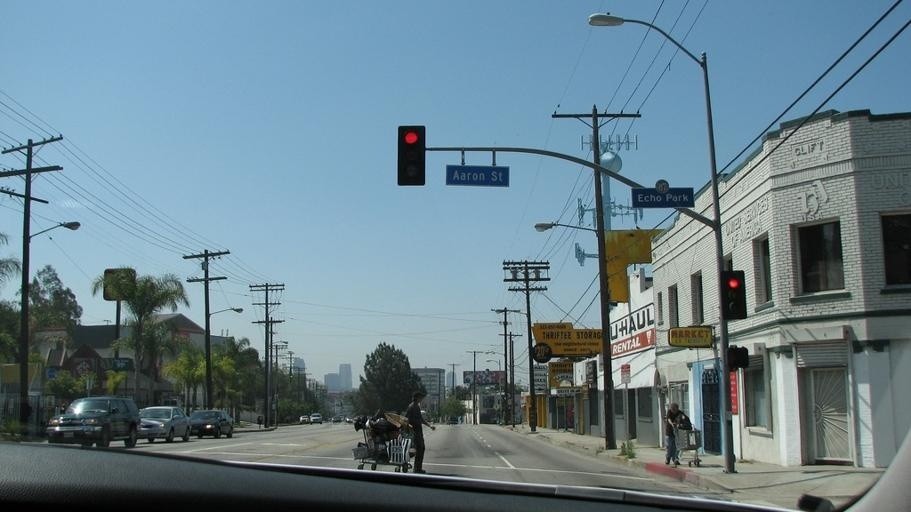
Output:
[534,219,617,454]
[271,341,287,346]
[485,358,503,423]
[21,220,81,436]
[205,307,244,410]
[586,12,739,470]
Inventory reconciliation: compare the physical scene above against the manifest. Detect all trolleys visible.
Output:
[358,425,415,474]
[668,421,704,468]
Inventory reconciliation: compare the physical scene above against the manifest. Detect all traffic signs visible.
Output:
[634,186,692,209]
[449,166,508,186]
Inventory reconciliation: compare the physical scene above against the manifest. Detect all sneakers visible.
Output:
[413,468,427,474]
[665,456,681,465]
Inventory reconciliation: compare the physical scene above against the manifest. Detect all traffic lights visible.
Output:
[729,275,743,313]
[738,346,751,371]
[403,133,419,181]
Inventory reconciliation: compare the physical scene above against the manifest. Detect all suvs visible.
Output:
[44,395,142,450]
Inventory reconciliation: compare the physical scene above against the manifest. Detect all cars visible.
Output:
[300,412,362,426]
[136,405,193,444]
[420,410,458,426]
[186,410,237,440]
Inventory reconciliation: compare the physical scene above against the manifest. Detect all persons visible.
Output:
[665,402,682,465]
[405,393,436,474]
